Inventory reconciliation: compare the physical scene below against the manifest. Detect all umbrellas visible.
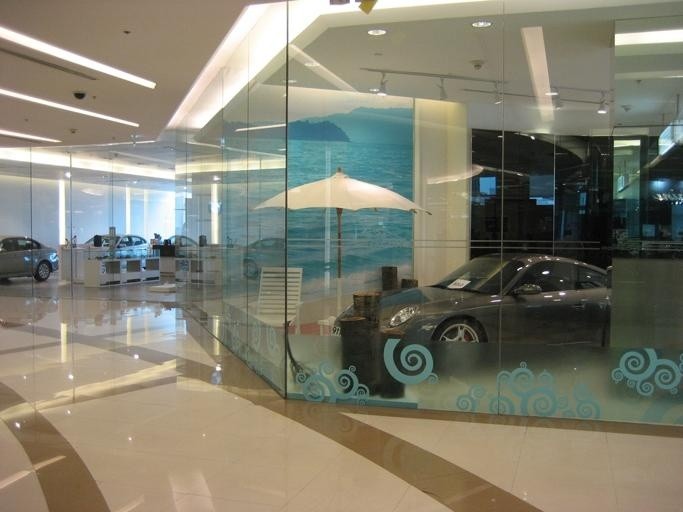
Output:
[251,166,431,316]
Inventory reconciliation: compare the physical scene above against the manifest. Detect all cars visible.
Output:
[0,236,59,282]
[82,234,149,258]
[240,238,284,279]
[330,252,611,364]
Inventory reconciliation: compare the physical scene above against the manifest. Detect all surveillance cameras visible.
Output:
[74,90,85,100]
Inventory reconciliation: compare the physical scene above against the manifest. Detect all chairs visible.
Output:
[254,267,305,336]
